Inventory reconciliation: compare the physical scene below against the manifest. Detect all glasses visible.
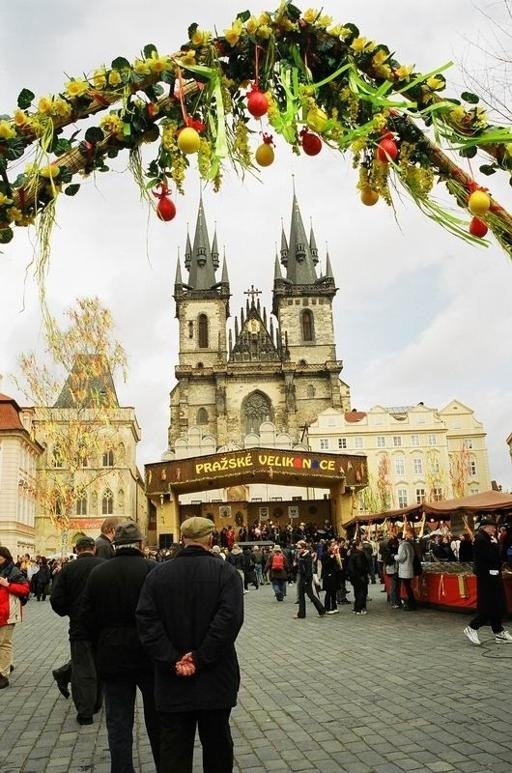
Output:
[297,547,303,549]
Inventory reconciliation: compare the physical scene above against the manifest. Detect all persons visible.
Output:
[71,523,162,772]
[50,537,112,725]
[0,547,30,689]
[463,518,511,645]
[52,518,121,699]
[14,521,511,617]
[135,516,243,772]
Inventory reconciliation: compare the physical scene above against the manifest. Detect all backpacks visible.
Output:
[271,554,285,570]
[20,576,32,605]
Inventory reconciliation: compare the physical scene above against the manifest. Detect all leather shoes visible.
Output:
[292,613,305,618]
[11,665,15,672]
[0,676,10,689]
[317,608,326,618]
[53,667,70,699]
[75,713,95,725]
[94,704,102,713]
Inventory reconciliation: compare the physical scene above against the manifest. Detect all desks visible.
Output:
[383,566,511,619]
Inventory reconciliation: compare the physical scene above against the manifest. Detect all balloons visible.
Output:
[302,108,327,156]
[158,196,176,221]
[361,188,379,206]
[379,137,398,162]
[467,190,490,237]
[178,128,200,154]
[248,92,274,166]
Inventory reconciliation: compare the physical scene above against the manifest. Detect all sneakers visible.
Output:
[480,517,497,525]
[293,600,300,605]
[352,608,368,615]
[325,609,339,614]
[389,604,400,609]
[464,625,481,644]
[495,631,512,644]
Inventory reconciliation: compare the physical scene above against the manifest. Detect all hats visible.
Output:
[110,520,148,545]
[180,516,216,538]
[77,536,97,549]
[273,544,282,551]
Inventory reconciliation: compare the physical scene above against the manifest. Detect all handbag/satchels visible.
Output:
[325,567,346,591]
[413,555,423,575]
